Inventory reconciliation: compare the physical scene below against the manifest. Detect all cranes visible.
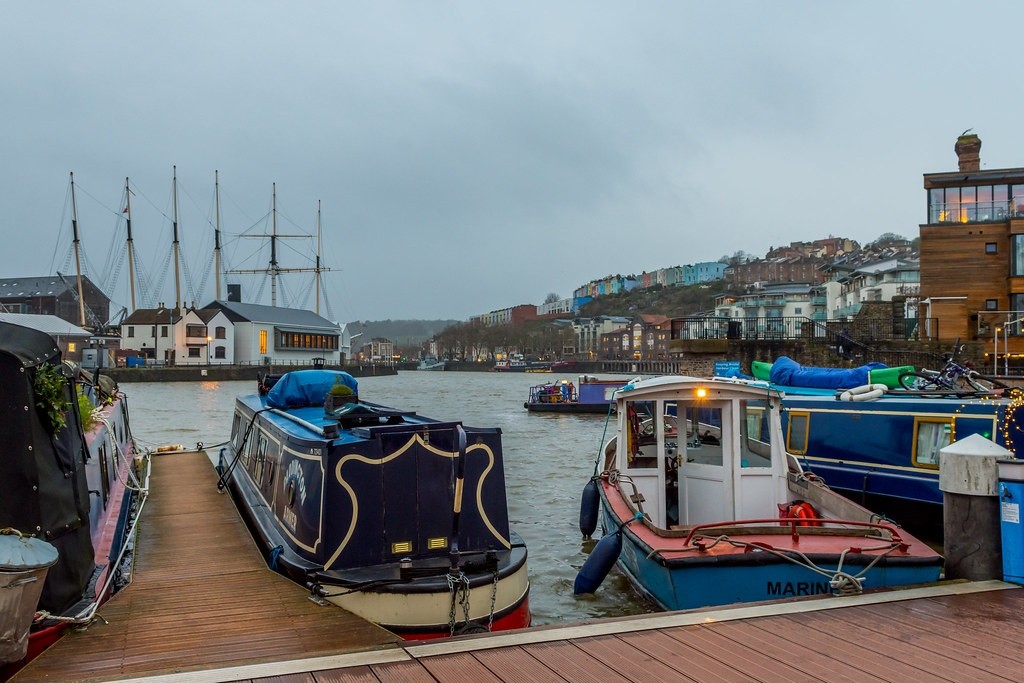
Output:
[92,307,128,332]
[56,271,120,337]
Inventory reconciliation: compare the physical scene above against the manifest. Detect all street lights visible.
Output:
[208,337,211,364]
[995,327,1001,375]
[1004,318,1024,376]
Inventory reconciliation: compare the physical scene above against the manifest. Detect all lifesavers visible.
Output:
[786,502,820,526]
[840,383,888,402]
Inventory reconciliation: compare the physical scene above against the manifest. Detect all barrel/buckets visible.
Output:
[0,528,59,664]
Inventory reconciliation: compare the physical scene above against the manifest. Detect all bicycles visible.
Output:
[898,337,1011,399]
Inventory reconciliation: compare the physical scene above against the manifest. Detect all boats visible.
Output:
[417,359,445,371]
[1,321,148,682]
[663,355,1024,512]
[524,374,632,413]
[595,375,946,611]
[217,369,532,642]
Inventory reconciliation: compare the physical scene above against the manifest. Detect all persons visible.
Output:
[560,381,574,404]
